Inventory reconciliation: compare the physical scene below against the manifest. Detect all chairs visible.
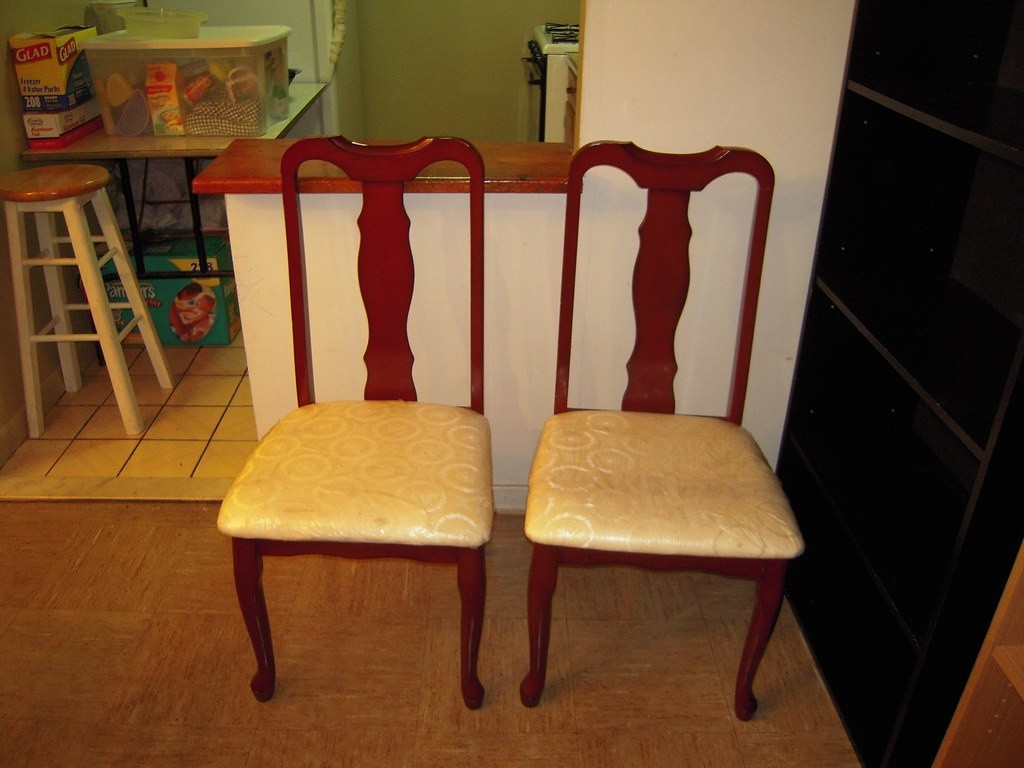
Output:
[519,138,807,722]
[213,135,497,712]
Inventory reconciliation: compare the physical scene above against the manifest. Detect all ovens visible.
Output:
[515,38,569,143]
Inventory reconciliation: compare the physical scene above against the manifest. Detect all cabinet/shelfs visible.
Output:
[777,0,1024,768]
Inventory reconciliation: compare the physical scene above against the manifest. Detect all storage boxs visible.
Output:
[8,24,104,152]
[78,235,242,346]
[79,25,294,137]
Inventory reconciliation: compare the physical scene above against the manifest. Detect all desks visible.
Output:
[21,82,327,366]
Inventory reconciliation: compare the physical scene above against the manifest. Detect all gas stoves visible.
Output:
[544,22,580,43]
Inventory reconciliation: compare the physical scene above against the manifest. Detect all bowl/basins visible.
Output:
[116,7,208,38]
[288,69,302,86]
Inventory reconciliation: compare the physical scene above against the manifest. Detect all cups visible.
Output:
[226,66,259,103]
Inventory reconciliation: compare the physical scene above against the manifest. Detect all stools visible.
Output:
[0,164,177,438]
